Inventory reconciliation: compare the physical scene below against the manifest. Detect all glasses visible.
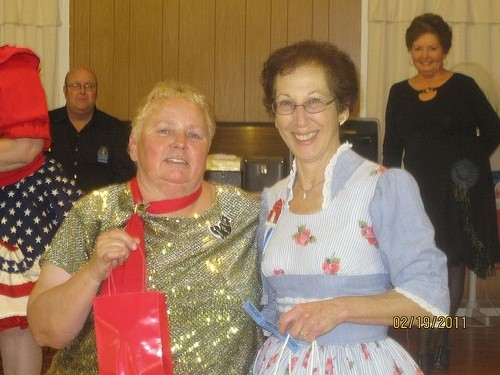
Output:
[67,83,95,90]
[271,99,337,115]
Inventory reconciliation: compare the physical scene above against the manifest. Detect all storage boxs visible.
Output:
[205,170,245,190]
[244,155,284,191]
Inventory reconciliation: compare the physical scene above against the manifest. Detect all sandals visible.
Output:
[434,345,451,369]
[418,353,433,375]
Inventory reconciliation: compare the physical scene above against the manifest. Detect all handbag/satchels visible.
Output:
[92,245,175,375]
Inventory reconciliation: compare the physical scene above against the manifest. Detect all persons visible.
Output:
[250,35,450,374]
[27,78,263,375]
[46,65,137,193]
[381,11,500,374]
[0,42,83,375]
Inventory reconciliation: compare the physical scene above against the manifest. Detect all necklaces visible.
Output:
[294,174,325,201]
